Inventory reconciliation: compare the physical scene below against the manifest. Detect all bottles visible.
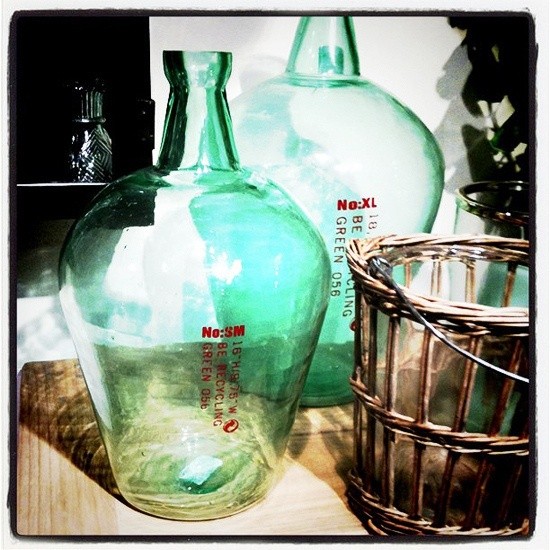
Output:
[67,83,117,220]
[201,19,443,406]
[57,50,330,521]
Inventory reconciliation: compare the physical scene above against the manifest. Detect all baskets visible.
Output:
[344,236,536,536]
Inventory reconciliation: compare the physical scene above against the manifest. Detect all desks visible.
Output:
[17,342,372,542]
